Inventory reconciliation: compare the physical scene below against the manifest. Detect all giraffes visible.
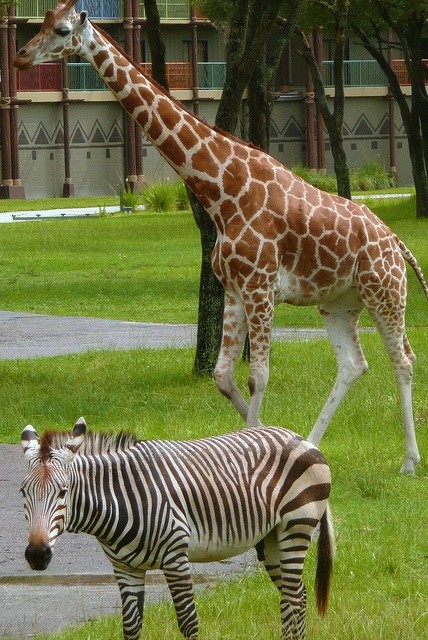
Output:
[11,1,428,480]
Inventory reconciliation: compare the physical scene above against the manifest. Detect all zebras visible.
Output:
[17,414,338,640]
